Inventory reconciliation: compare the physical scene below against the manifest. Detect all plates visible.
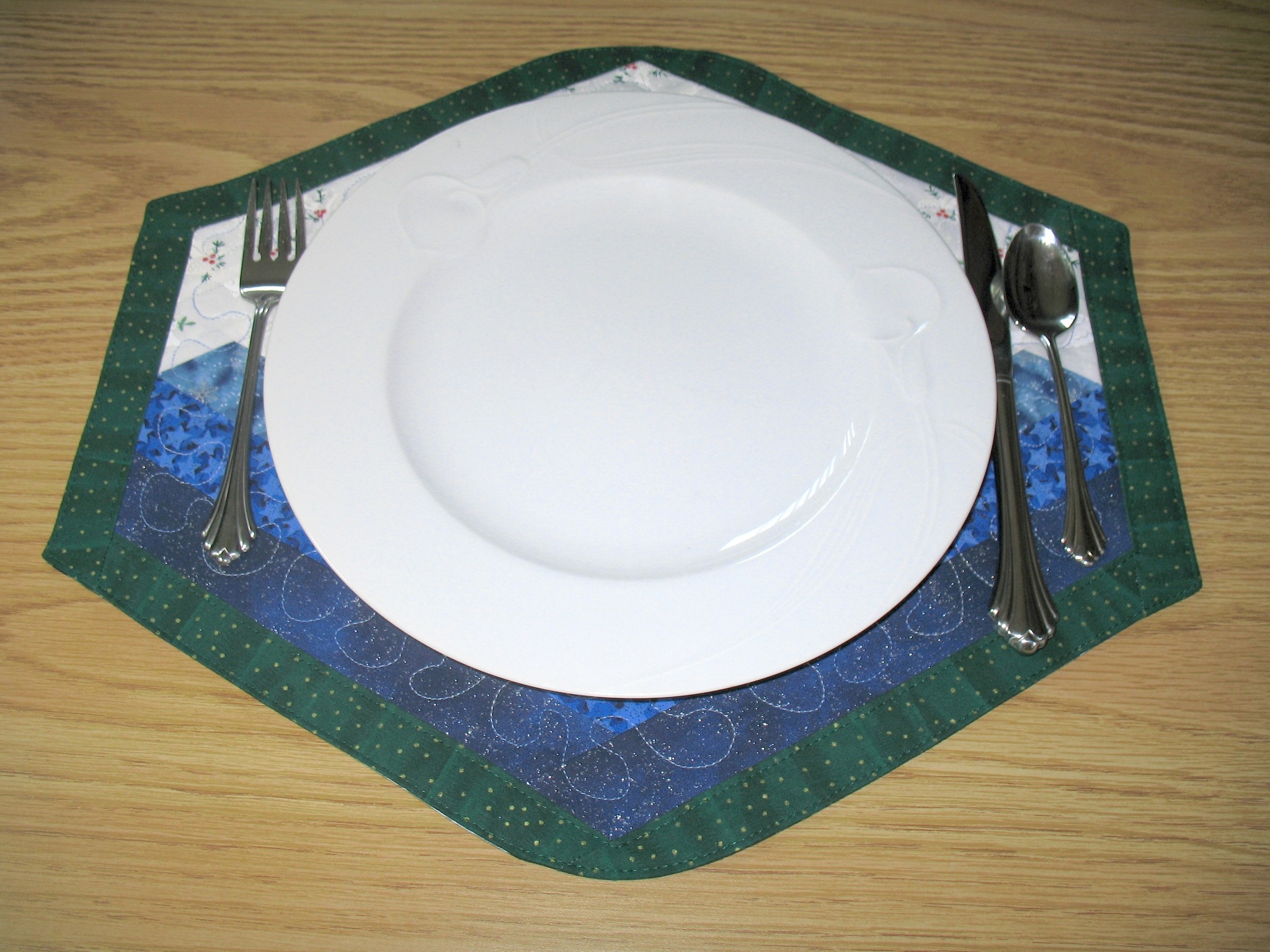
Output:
[262,91,997,701]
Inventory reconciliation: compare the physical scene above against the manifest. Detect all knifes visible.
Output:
[952,171,1061,656]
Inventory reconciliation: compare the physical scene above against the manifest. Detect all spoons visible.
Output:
[1004,221,1108,567]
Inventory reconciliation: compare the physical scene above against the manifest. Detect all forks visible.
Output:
[200,177,306,567]
